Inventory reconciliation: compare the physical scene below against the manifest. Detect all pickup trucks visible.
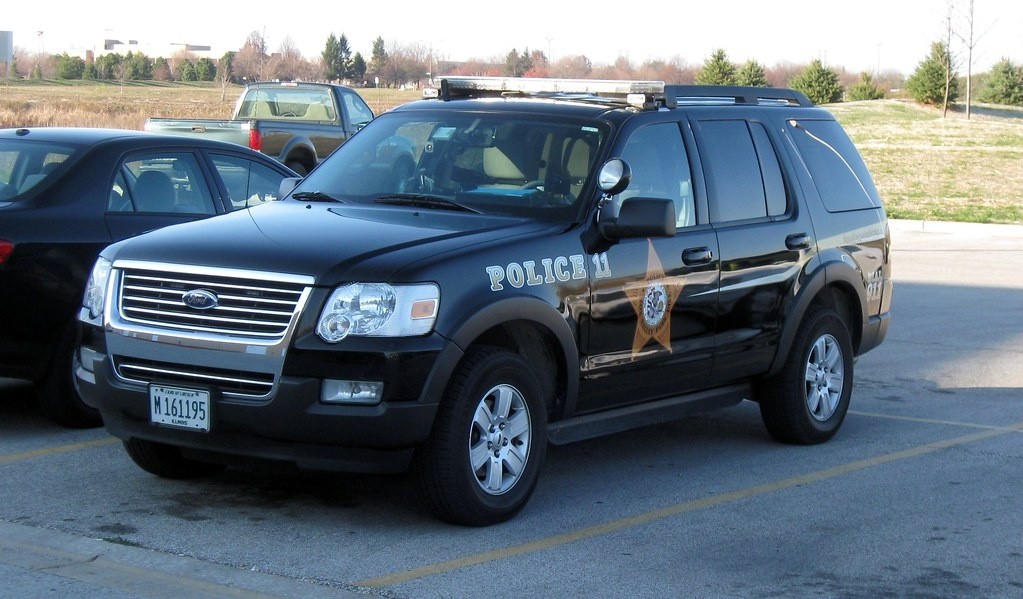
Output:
[143,80,418,196]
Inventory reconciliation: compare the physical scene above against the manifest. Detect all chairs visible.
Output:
[474,136,538,204]
[301,101,331,121]
[120,172,177,213]
[250,98,277,119]
[606,141,690,226]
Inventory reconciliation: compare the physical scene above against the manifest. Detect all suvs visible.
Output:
[71,76,892,524]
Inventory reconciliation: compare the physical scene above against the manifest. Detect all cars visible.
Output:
[0,125,306,431]
